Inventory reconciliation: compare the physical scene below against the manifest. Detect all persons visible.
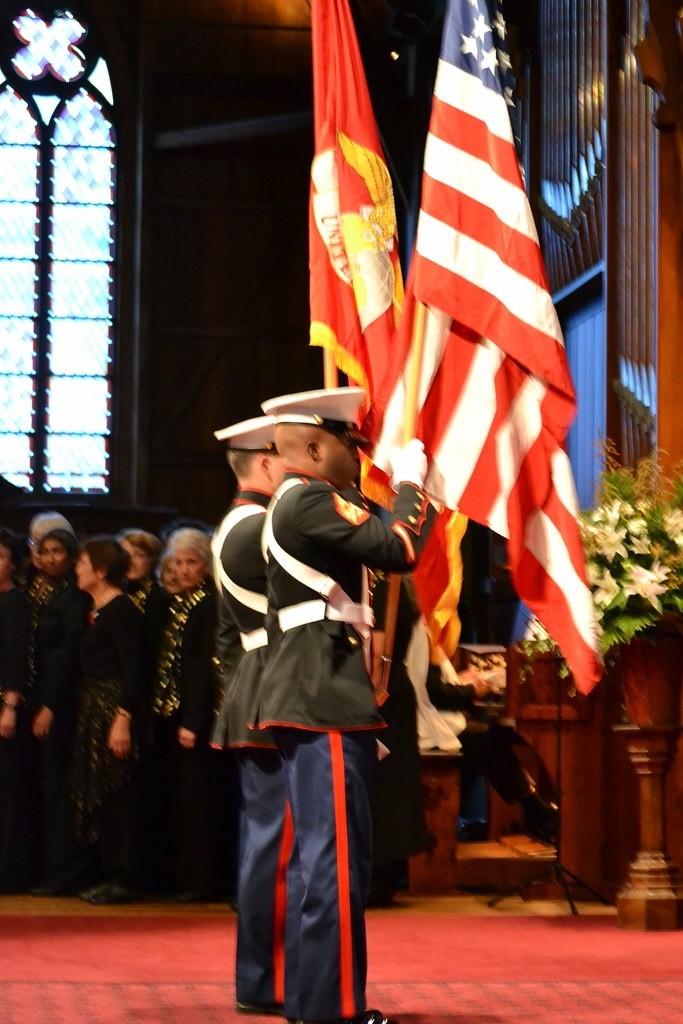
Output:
[214,386,437,1024]
[372,581,493,889]
[0,514,243,907]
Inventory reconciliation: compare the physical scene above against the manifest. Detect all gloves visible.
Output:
[386,439,429,494]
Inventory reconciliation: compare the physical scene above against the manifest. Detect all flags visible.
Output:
[299,0,605,697]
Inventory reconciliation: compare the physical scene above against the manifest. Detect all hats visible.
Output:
[259,388,373,451]
[213,415,280,455]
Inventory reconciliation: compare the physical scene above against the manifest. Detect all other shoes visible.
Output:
[80,876,142,903]
[236,1000,287,1015]
[27,867,77,896]
[175,889,197,905]
[287,1007,388,1024]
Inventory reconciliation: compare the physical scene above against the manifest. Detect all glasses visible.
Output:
[27,536,41,550]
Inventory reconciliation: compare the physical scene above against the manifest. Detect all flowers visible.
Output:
[522,429,683,698]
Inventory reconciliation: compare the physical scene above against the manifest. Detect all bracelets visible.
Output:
[115,709,131,721]
